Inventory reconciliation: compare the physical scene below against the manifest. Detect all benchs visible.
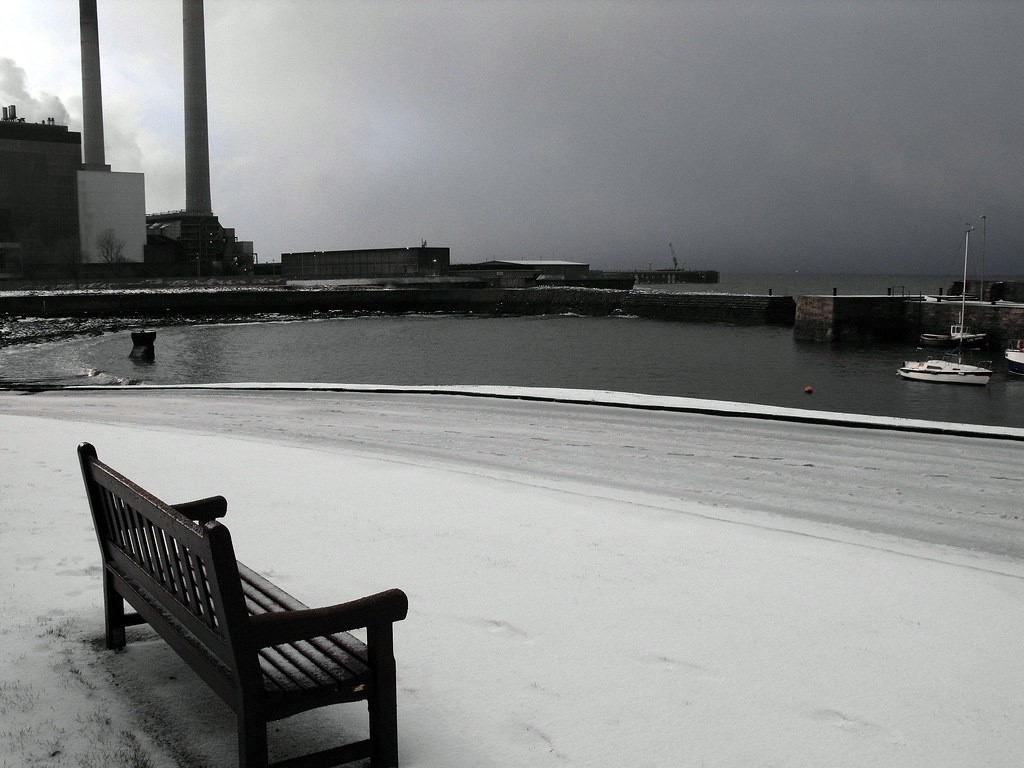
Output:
[76,442,408,768]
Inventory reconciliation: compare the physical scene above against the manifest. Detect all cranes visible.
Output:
[668,241,679,271]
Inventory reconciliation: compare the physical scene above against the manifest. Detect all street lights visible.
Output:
[979,214,986,301]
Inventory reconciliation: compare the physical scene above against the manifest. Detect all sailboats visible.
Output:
[895,231,996,388]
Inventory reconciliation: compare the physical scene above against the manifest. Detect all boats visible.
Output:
[130,329,157,346]
[1004,338,1024,377]
[918,309,987,352]
